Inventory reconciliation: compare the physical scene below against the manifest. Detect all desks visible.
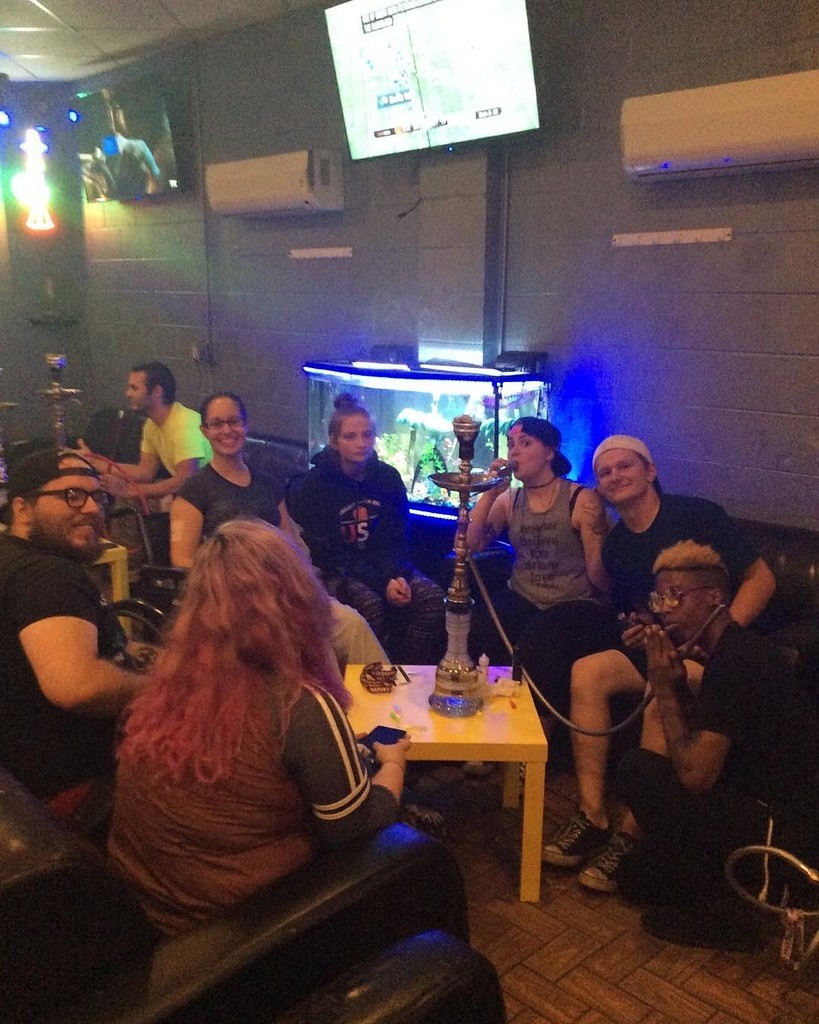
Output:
[91,538,132,637]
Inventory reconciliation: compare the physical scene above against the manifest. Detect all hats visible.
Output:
[0,447,101,524]
[507,417,572,477]
[592,434,653,474]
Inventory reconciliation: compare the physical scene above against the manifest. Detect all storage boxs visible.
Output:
[303,359,553,565]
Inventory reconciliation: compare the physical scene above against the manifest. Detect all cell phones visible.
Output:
[356,726,406,764]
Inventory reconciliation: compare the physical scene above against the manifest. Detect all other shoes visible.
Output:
[518,737,572,783]
[639,884,759,953]
[463,760,497,775]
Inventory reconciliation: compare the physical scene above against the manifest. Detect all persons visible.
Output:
[0,391,389,843]
[104,519,472,975]
[60,361,212,513]
[286,393,448,663]
[453,417,819,947]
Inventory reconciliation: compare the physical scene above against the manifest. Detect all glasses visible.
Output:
[16,486,115,511]
[202,416,247,430]
[647,585,728,614]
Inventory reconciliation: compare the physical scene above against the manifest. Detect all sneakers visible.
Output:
[542,807,612,867]
[577,833,635,894]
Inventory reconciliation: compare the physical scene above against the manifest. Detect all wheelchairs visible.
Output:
[94,503,191,675]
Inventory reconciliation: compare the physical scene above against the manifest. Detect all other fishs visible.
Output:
[395,402,454,438]
[480,392,533,408]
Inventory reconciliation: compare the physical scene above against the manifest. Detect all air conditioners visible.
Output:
[621,69,819,182]
[206,148,344,217]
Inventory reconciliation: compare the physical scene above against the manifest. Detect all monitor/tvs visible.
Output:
[68,78,189,204]
[325,0,543,164]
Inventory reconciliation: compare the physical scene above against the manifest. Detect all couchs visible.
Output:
[433,517,819,673]
[0,766,506,1024]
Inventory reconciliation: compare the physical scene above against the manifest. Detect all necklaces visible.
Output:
[525,477,556,489]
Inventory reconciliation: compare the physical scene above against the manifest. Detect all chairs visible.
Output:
[286,472,307,526]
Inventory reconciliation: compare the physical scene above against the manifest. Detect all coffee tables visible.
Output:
[341,663,548,903]
